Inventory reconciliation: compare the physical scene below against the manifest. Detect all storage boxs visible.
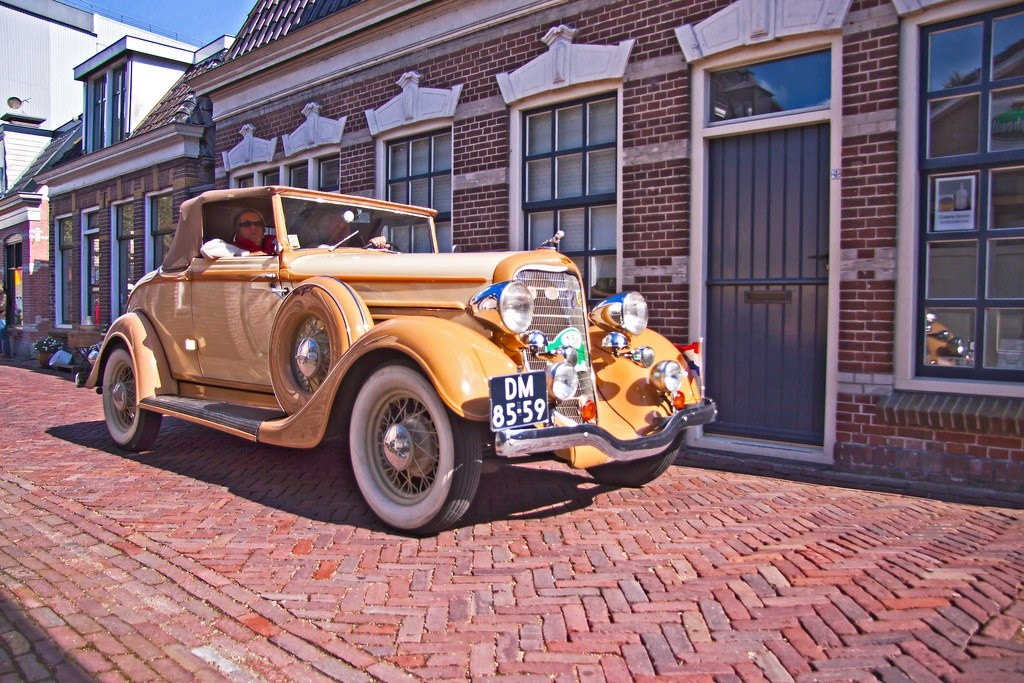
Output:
[68,331,101,346]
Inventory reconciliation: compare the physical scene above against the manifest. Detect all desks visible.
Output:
[69,345,93,371]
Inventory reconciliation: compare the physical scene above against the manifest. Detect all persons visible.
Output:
[200,207,269,260]
[320,213,391,248]
[0,280,6,331]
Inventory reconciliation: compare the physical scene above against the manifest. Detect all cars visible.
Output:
[75,185,717,537]
[927,315,976,368]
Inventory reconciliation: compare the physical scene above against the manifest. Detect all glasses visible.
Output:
[238,220,263,226]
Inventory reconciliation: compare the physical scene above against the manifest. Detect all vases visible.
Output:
[37,351,57,368]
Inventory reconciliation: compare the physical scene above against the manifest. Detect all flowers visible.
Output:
[89,341,104,352]
[33,334,64,355]
